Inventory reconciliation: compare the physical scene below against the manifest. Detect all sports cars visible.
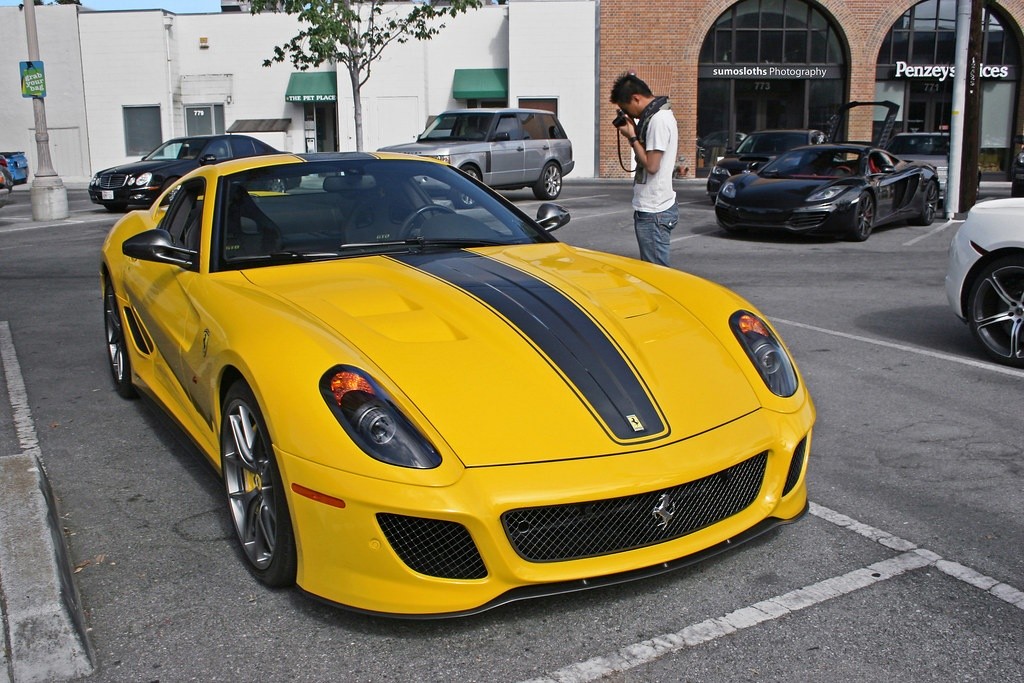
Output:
[101,150,818,623]
[714,142,942,242]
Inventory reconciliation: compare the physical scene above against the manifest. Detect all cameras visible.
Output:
[612,109,627,128]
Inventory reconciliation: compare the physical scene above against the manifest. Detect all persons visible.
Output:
[610,71,679,268]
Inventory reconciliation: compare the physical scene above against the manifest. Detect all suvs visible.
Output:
[945,197,1024,369]
[886,132,950,199]
[706,128,828,203]
[376,108,576,202]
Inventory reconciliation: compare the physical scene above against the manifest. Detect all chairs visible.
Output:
[223,201,310,260]
[801,156,888,177]
[342,196,425,253]
[214,147,227,160]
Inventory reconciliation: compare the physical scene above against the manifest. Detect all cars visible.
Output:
[1012,145,1024,200]
[87,133,301,213]
[0,152,30,210]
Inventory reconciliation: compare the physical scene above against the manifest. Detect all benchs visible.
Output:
[184,176,380,240]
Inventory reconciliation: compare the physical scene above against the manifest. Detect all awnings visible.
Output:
[285,71,337,102]
[453,68,508,99]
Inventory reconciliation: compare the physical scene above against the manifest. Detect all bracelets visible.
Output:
[628,137,637,147]
